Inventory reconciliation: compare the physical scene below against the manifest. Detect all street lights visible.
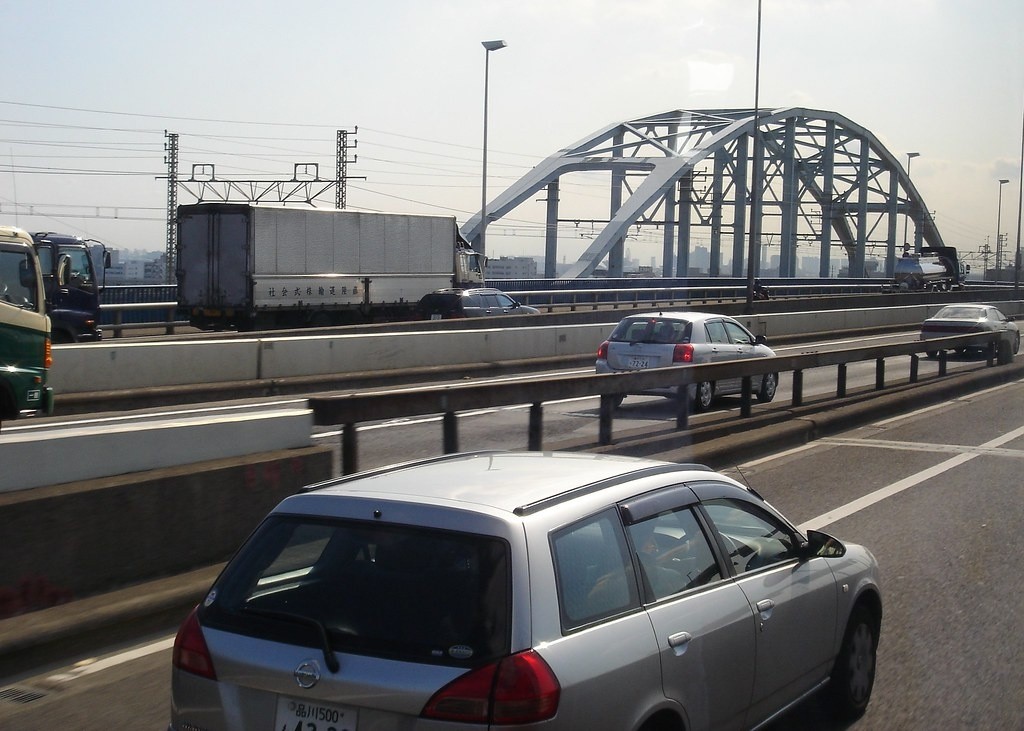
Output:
[994,179,1010,286]
[903,152,919,253]
[483,41,506,277]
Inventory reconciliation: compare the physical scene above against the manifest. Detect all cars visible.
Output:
[920,305,1021,359]
[596,311,779,411]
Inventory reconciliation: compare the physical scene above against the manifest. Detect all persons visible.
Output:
[629,515,702,593]
[816,538,842,556]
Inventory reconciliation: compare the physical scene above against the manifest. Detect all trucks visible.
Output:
[30,231,110,342]
[176,204,489,330]
[0,227,55,419]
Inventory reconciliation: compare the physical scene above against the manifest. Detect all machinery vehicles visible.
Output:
[894,246,970,291]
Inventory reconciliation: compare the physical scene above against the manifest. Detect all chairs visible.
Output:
[659,326,675,343]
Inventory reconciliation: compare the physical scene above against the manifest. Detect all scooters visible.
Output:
[754,288,769,300]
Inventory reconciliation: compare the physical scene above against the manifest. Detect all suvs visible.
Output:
[411,287,541,320]
[170,450,885,730]
